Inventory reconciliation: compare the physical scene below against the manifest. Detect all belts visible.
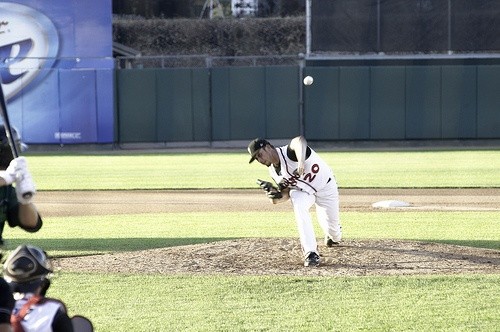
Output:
[327,177,332,183]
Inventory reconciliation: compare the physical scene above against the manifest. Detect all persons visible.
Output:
[4,245,94,332]
[0,128,43,332]
[247,135,343,266]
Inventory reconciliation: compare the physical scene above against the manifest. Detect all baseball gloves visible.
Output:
[258,180,285,199]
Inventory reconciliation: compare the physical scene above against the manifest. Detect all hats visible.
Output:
[248,138,267,164]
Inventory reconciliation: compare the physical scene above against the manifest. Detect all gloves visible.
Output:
[0,156,28,185]
[15,171,35,205]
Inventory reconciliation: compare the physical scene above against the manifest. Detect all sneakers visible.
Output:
[327,236,339,247]
[304,252,320,266]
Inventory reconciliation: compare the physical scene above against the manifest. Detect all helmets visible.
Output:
[0,125,28,152]
[4,245,52,292]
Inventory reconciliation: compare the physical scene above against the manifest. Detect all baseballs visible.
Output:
[303,76,314,86]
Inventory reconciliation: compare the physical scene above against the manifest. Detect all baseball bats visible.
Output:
[0,83,34,200]
